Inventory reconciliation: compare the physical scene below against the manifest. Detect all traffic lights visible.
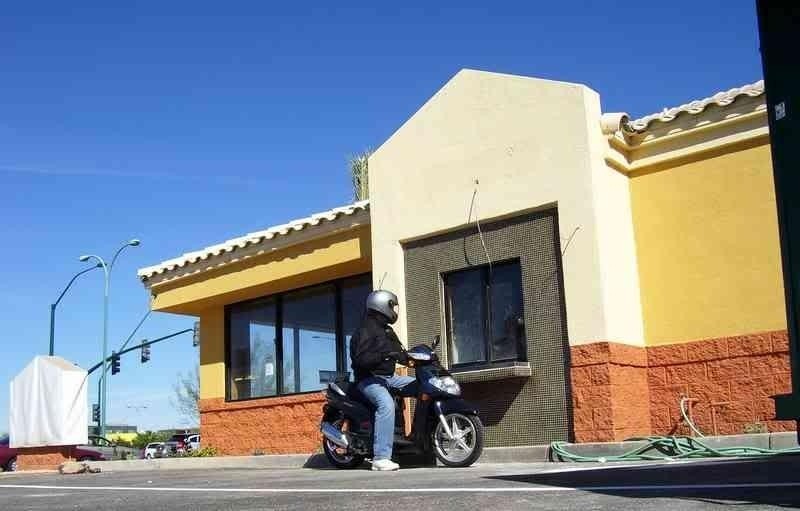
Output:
[140,336,151,364]
[92,401,101,422]
[111,348,121,376]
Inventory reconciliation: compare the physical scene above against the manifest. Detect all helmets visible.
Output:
[366,290,399,325]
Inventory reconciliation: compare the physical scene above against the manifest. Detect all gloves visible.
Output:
[384,351,400,360]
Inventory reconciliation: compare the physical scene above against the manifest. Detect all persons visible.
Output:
[350,290,422,472]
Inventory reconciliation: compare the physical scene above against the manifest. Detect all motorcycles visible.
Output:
[315,332,489,469]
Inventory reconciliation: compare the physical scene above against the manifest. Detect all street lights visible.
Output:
[310,334,336,341]
[78,236,142,445]
[48,257,109,356]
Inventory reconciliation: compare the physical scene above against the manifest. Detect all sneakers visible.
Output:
[371,459,400,471]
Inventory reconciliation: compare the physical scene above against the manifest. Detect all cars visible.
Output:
[0,431,200,473]
